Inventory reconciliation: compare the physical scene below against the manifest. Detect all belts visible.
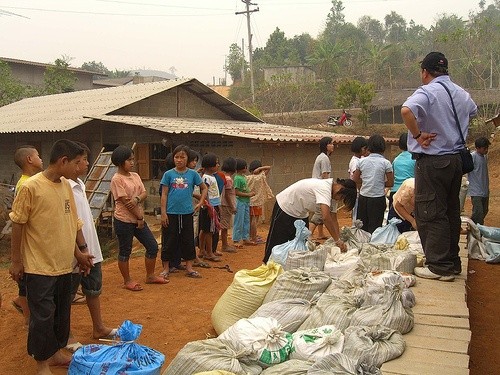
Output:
[412,153,432,159]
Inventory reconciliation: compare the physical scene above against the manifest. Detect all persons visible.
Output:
[304,137,334,241]
[466,137,491,225]
[347,137,373,229]
[161,150,238,273]
[159,145,207,280]
[263,178,357,265]
[388,177,418,234]
[388,133,417,209]
[233,159,256,248]
[244,160,274,245]
[400,52,478,280]
[10,139,122,375]
[111,146,170,290]
[354,134,394,235]
[340,110,346,126]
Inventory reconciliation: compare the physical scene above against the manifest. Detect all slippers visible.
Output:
[198,253,207,257]
[92,329,119,338]
[23,324,29,331]
[177,265,186,270]
[203,255,223,261]
[11,300,23,314]
[187,271,201,278]
[48,358,71,368]
[65,342,82,354]
[317,235,330,240]
[159,272,169,279]
[192,261,211,268]
[71,296,87,304]
[145,276,169,283]
[122,282,143,291]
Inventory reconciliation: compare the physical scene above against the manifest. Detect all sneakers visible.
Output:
[414,266,455,280]
[449,268,461,273]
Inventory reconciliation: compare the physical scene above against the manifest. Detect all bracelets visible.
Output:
[78,243,87,250]
[335,238,340,244]
[135,196,141,203]
[137,217,143,220]
[412,130,422,139]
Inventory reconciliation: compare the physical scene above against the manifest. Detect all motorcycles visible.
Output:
[326,104,353,128]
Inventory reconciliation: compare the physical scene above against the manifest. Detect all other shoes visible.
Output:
[169,267,179,273]
[243,242,257,246]
[214,251,223,255]
[233,244,244,249]
[222,247,236,251]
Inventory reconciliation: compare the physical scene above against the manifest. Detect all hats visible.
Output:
[419,52,449,69]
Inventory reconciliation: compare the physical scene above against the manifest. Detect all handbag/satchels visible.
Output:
[459,147,474,174]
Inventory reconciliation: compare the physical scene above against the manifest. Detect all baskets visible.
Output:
[345,114,352,119]
